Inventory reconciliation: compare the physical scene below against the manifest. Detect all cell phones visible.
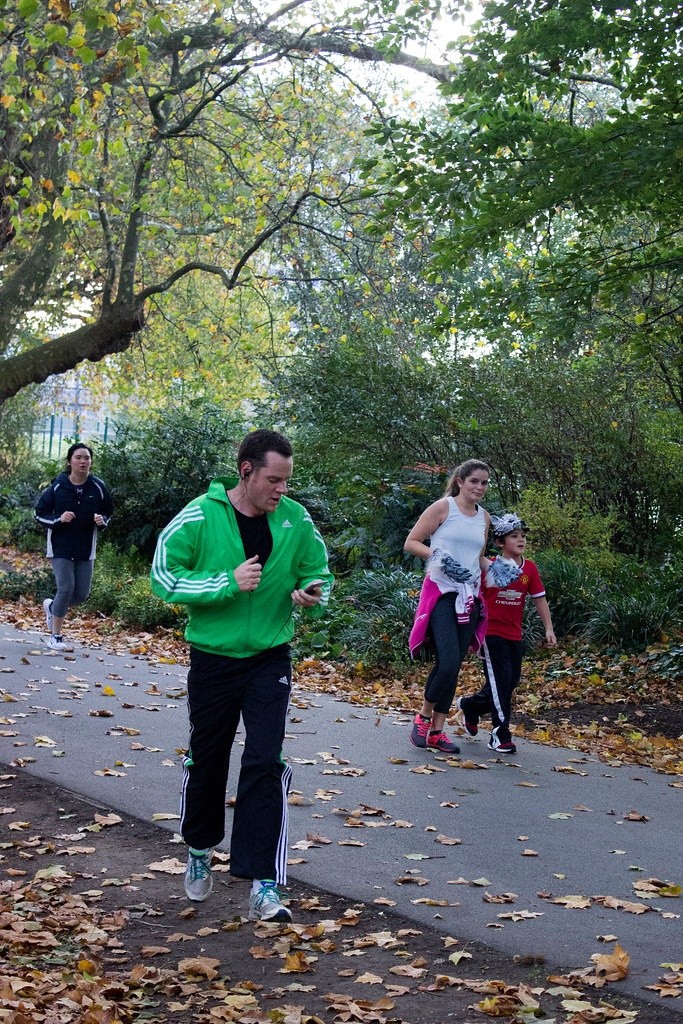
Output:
[305,581,327,595]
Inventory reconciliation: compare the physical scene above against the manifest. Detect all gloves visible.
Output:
[432,547,472,583]
[487,556,523,588]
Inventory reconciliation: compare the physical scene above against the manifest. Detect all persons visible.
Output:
[149,429,334,922]
[32,442,115,651]
[403,460,523,754]
[456,515,557,754]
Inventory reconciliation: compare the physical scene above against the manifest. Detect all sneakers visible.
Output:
[409,713,430,748]
[487,741,516,753]
[43,599,54,631]
[47,634,66,651]
[184,846,214,902]
[247,878,293,923]
[427,732,460,753]
[456,697,478,736]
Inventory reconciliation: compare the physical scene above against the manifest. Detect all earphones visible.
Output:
[242,470,251,478]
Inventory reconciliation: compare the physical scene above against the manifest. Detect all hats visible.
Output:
[490,512,530,538]
